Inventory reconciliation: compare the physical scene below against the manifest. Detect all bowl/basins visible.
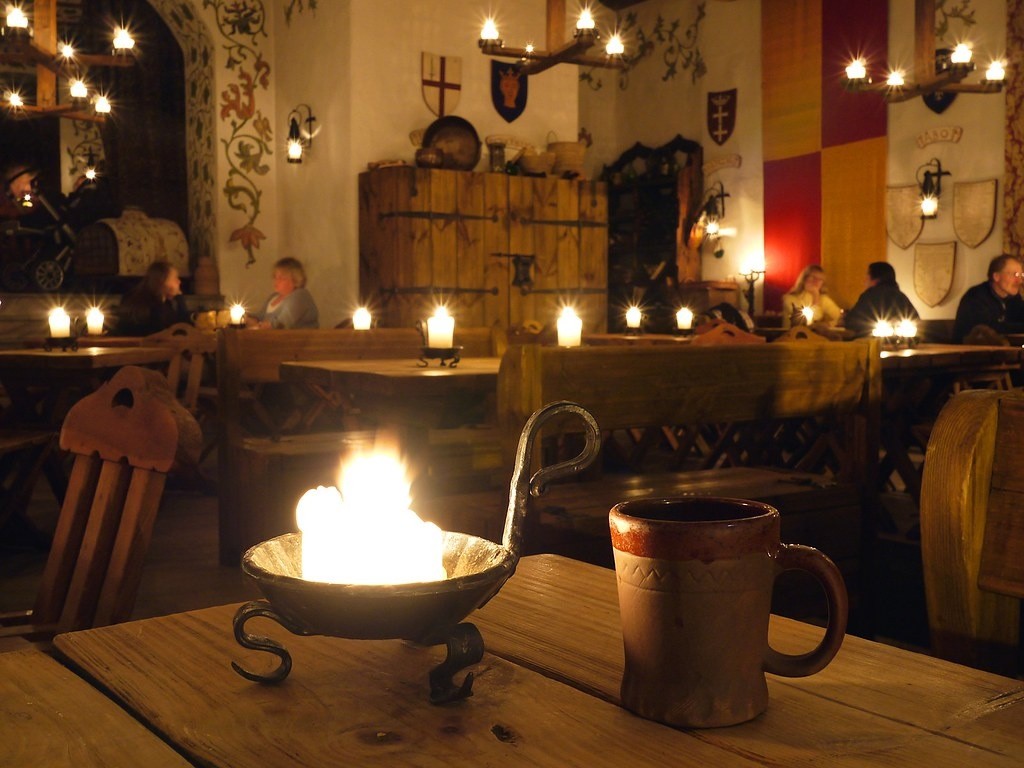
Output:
[520,155,546,177]
[415,149,442,168]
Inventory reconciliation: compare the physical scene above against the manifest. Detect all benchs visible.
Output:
[0,324,1024,680]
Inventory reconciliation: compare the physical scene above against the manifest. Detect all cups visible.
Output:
[191,308,217,329]
[610,494,848,727]
[216,309,231,328]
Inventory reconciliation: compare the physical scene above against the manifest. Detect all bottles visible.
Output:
[195,258,218,296]
[486,136,507,173]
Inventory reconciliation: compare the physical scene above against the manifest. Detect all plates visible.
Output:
[425,116,482,170]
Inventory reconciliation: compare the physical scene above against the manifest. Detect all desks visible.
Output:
[755,327,858,342]
[0,551,1024,768]
[877,342,1024,510]
[0,345,174,513]
[24,333,149,348]
[579,330,695,346]
[279,357,505,442]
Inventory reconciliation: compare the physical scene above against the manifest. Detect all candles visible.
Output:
[230,304,246,324]
[294,440,448,587]
[676,307,693,329]
[625,306,642,329]
[85,307,104,336]
[557,306,582,348]
[428,306,455,348]
[48,306,71,337]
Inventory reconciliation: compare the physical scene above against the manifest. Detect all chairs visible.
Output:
[0,322,218,654]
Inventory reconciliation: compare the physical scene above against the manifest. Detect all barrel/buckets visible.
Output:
[548,132,593,180]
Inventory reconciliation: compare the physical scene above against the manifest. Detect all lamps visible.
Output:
[702,180,731,236]
[286,104,317,163]
[476,0,626,74]
[0,0,137,124]
[916,158,951,220]
[82,146,106,184]
[840,0,1003,104]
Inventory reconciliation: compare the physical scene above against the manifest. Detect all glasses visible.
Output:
[994,271,1024,280]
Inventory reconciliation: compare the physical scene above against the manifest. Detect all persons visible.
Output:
[104,262,195,336]
[783,264,842,329]
[238,257,319,330]
[846,262,921,344]
[951,254,1024,366]
